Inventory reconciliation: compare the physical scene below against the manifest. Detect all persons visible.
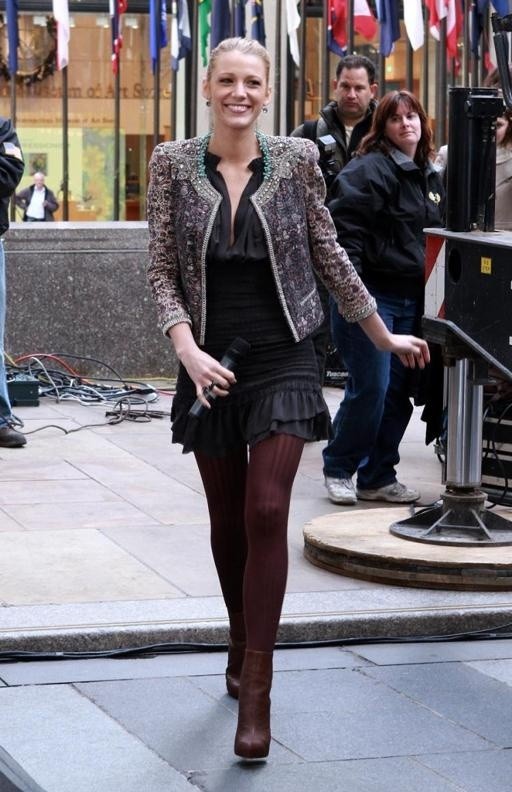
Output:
[13,170,58,222]
[321,89,445,505]
[145,35,432,761]
[288,50,384,394]
[0,60,26,448]
[431,61,512,233]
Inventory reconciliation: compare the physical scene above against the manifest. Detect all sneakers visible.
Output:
[323,469,359,506]
[357,478,422,503]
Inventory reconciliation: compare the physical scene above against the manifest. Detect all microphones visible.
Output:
[188,336,251,417]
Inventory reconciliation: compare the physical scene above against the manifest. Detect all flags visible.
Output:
[328,1,510,76]
[9,0,303,79]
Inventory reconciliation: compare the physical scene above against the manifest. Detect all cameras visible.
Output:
[317,133,337,187]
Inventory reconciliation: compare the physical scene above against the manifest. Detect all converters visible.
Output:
[130,385,153,393]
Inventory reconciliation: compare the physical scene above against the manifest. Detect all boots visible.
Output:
[233,648,279,762]
[220,611,246,696]
